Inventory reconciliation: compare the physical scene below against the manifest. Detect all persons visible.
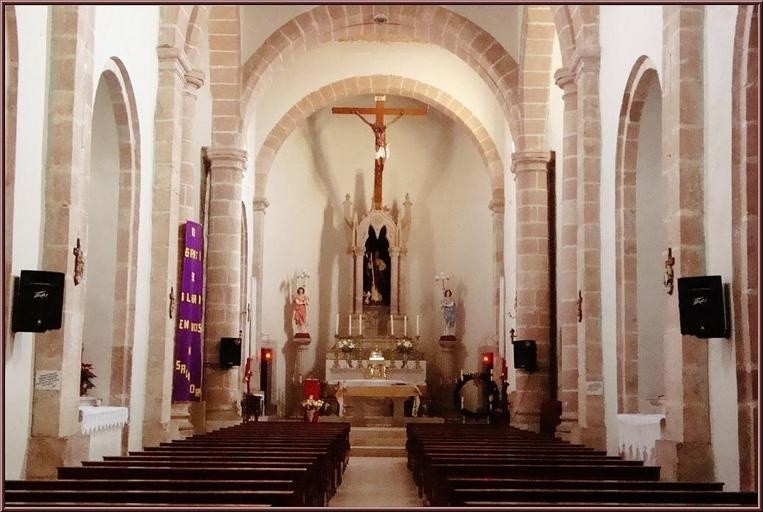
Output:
[367,251,387,306]
[295,288,309,332]
[352,111,404,175]
[440,290,455,336]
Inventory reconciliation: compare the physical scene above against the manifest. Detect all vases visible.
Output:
[401,352,409,368]
[343,352,352,369]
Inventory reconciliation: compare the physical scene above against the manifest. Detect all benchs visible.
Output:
[406,423,757,506]
[4,422,351,507]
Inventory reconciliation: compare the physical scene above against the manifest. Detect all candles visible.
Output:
[404,316,407,336]
[391,314,394,335]
[349,315,352,337]
[359,314,362,335]
[336,314,339,335]
[416,315,419,336]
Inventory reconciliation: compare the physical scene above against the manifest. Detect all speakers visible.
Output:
[514,339,537,370]
[13,270,65,333]
[219,337,242,367]
[677,275,727,340]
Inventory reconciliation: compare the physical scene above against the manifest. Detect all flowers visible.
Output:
[337,339,354,351]
[396,339,413,353]
